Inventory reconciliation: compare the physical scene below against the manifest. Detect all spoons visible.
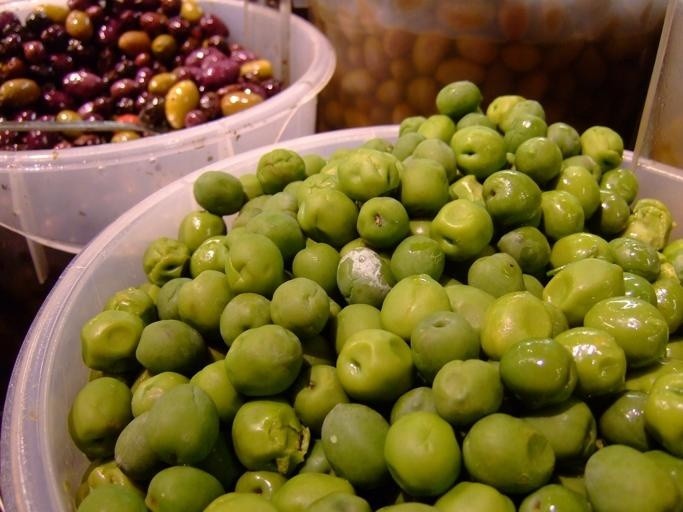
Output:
[0,109,175,136]
